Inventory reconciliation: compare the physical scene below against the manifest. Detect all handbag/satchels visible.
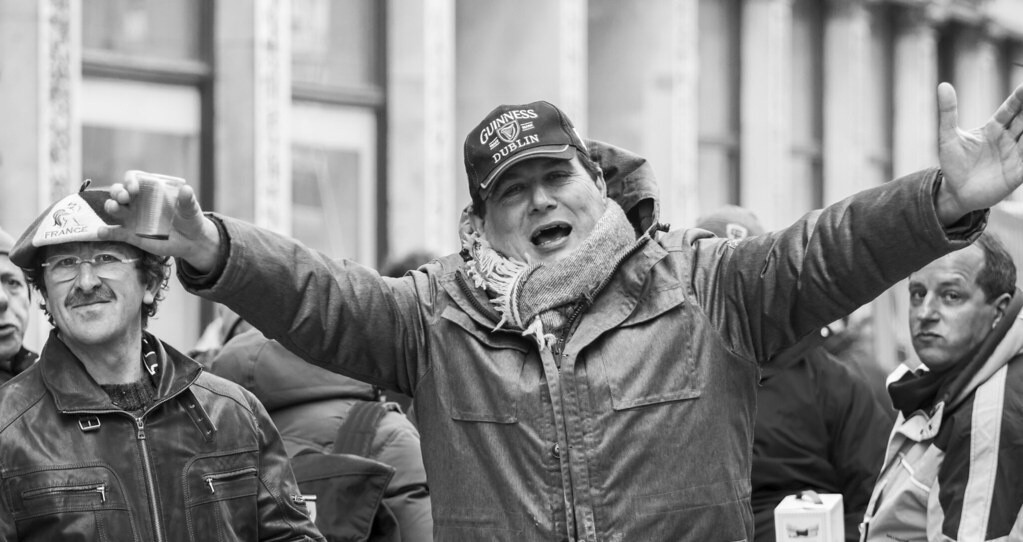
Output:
[289,399,395,542]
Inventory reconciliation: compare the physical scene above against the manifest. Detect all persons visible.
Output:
[0,229,41,383]
[188,250,456,542]
[105,82,1023,542]
[0,193,325,542]
[692,205,894,542]
[860,231,1023,542]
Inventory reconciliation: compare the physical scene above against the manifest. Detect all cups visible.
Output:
[134,171,186,240]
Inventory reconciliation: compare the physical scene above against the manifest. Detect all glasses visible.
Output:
[40,251,141,285]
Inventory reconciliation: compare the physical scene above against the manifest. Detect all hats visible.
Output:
[8,179,166,268]
[0,228,15,253]
[464,100,590,202]
[692,204,766,239]
